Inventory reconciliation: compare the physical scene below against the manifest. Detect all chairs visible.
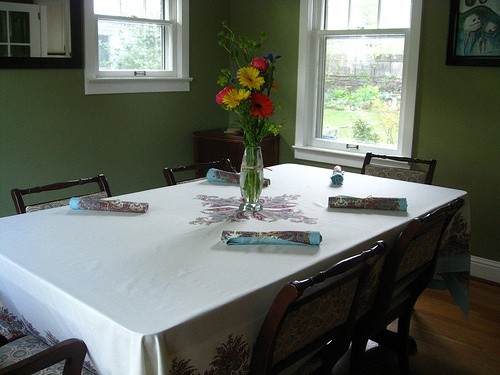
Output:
[0,150,438,375]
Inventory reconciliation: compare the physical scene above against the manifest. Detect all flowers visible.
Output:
[213,18,286,203]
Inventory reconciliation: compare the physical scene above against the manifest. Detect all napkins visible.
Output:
[222,230,323,247]
[69,195,149,213]
[207,167,271,188]
[328,194,408,213]
[331,165,344,185]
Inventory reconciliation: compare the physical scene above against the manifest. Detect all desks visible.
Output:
[0,163,473,375]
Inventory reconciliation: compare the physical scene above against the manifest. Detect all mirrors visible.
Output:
[0,0,83,69]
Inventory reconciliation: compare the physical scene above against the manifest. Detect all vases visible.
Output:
[238,145,265,212]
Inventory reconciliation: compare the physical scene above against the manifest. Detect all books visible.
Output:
[224,124,242,133]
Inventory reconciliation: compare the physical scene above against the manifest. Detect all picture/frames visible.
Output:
[444,0,500,68]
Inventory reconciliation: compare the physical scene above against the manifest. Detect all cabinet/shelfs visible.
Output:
[195,122,279,179]
